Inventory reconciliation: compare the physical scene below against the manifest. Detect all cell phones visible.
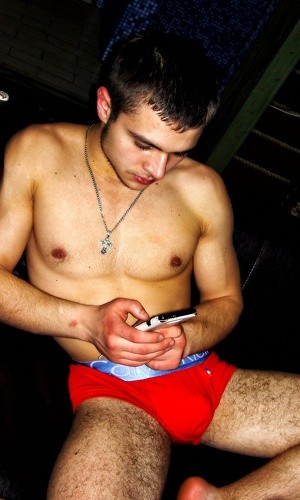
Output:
[133,307,197,333]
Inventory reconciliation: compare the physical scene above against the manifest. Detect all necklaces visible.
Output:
[84,123,146,256]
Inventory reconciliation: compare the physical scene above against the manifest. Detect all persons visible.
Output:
[1,35,300,500]
